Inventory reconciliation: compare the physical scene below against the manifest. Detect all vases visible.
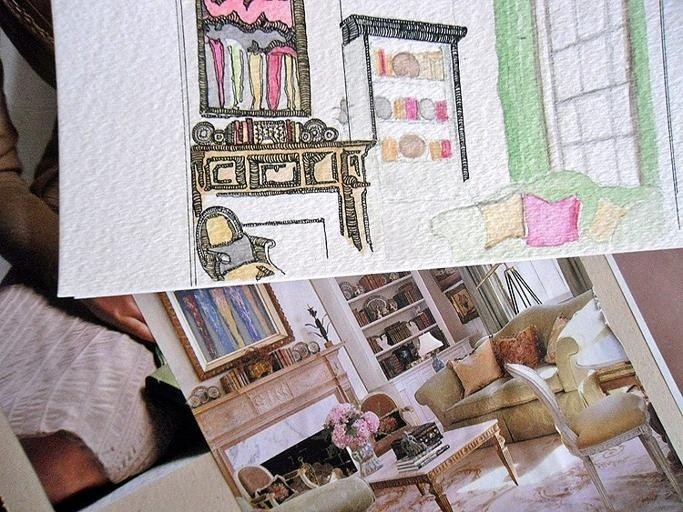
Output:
[351,446,382,478]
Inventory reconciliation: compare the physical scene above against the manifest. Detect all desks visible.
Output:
[190,342,359,506]
[191,140,373,252]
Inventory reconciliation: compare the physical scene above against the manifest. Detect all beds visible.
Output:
[353,418,522,512]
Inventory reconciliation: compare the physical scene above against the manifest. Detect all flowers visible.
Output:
[325,403,383,466]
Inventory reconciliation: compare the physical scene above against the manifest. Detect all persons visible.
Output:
[0,63,165,504]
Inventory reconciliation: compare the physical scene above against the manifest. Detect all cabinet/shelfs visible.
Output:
[342,10,473,200]
[310,271,453,380]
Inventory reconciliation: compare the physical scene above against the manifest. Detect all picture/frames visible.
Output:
[157,280,298,382]
[195,0,313,120]
[429,267,480,325]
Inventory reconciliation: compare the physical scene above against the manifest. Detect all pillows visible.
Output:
[211,236,260,275]
[478,192,628,248]
[253,474,299,504]
[445,312,573,400]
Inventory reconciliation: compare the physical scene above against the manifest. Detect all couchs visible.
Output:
[414,290,605,445]
[424,167,665,263]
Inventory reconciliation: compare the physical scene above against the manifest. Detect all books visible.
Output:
[220,274,451,474]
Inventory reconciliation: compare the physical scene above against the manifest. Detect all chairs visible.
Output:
[194,205,284,283]
[499,358,683,511]
[233,462,304,506]
